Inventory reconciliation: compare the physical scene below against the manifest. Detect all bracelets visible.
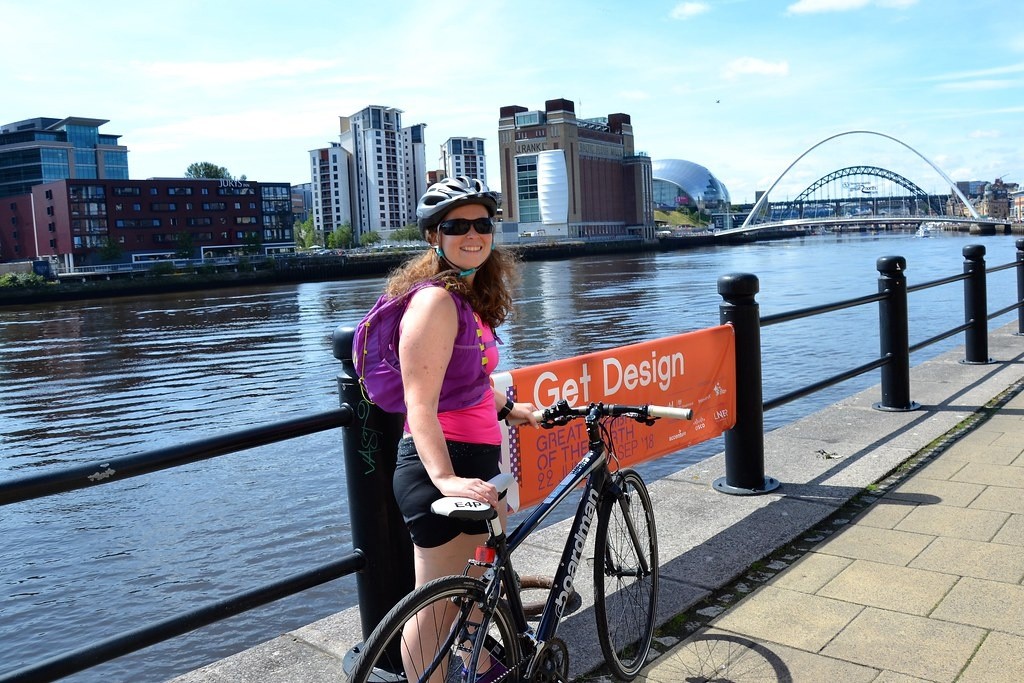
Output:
[497,399,514,421]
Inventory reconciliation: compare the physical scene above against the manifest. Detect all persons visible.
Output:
[392,174,539,683]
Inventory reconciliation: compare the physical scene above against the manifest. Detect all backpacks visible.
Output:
[351,282,489,416]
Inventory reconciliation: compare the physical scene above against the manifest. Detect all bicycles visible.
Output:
[341,403,695,683]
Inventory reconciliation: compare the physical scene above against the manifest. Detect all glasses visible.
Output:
[430,218,494,236]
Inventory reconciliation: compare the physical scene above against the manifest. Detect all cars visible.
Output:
[300,244,421,257]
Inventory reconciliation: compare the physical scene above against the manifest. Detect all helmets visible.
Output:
[415,175,500,236]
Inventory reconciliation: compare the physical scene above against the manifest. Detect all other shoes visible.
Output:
[461,654,506,683]
